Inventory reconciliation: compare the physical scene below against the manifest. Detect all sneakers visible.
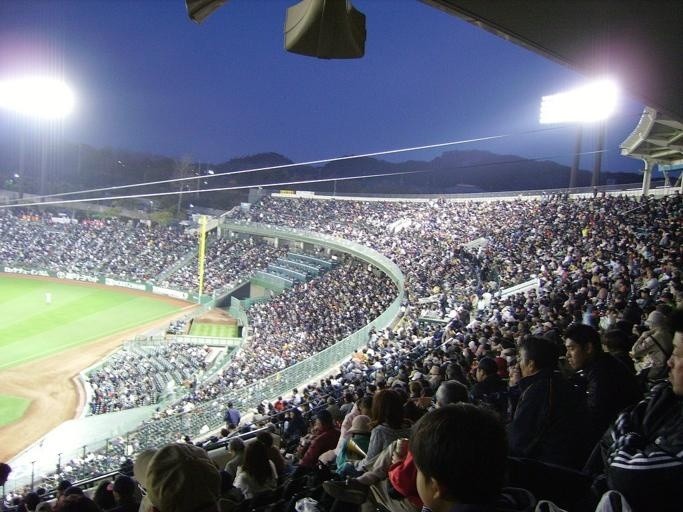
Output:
[320,477,369,505]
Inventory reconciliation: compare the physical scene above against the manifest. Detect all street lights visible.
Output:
[538,78,617,186]
[0,78,71,200]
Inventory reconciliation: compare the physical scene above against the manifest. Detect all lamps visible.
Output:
[282,0,366,61]
[185,1,228,27]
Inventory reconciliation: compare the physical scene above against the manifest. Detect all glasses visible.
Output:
[136,483,147,496]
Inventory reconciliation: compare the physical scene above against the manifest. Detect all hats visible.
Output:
[105,474,134,496]
[131,442,222,510]
[642,309,667,328]
[345,415,373,435]
[470,356,498,372]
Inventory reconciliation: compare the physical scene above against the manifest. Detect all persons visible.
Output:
[0,455,85,512]
[85,302,683,512]
[1,190,682,302]
